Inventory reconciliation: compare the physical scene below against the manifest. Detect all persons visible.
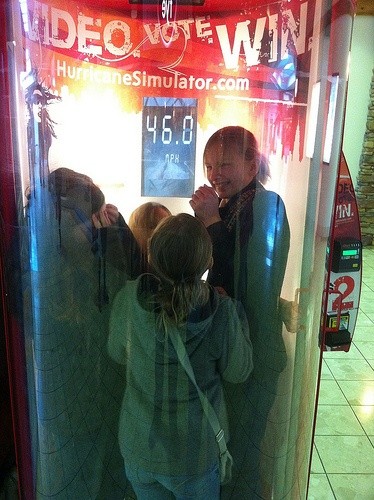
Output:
[107,212,255,499]
[189,127,289,500]
[22,47,62,185]
[130,203,172,259]
[21,168,144,499]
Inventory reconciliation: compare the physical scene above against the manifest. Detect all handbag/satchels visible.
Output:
[220,450,232,485]
